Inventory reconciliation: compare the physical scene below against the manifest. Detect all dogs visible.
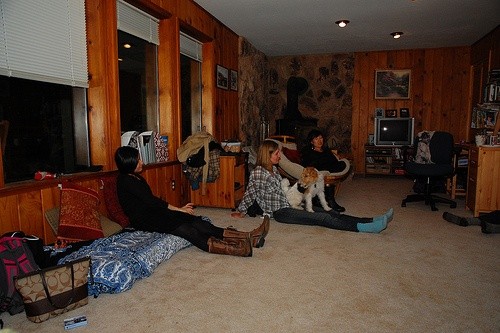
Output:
[282,167,332,213]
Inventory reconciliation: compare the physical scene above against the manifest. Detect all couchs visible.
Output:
[268,135,353,201]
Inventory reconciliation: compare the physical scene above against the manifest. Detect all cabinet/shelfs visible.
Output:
[189,152,249,212]
[364,143,414,178]
[446,141,500,217]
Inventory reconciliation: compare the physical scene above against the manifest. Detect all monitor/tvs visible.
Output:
[374,118,414,146]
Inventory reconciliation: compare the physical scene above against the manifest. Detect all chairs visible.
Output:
[400,129,462,212]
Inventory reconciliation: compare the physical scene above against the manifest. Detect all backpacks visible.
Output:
[0,236,43,315]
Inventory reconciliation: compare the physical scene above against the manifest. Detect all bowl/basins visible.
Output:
[221,143,227,149]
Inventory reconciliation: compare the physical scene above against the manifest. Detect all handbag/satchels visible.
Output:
[13,256,94,323]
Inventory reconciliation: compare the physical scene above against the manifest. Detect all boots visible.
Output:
[208,232,254,257]
[223,215,269,248]
[324,183,345,212]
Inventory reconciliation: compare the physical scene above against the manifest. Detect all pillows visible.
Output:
[57,180,104,241]
[103,181,130,228]
[45,207,122,238]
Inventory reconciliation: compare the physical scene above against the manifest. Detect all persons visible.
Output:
[236,141,393,233]
[114,146,270,257]
[299,130,346,210]
[443,210,500,234]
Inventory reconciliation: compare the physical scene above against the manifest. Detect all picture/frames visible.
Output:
[216,64,230,91]
[375,108,384,118]
[230,68,239,92]
[374,69,412,100]
[385,109,397,118]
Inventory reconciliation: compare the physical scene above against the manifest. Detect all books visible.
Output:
[470,82,500,128]
[121,132,154,165]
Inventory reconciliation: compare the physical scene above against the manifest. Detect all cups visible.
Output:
[490,136,497,145]
[475,136,486,146]
